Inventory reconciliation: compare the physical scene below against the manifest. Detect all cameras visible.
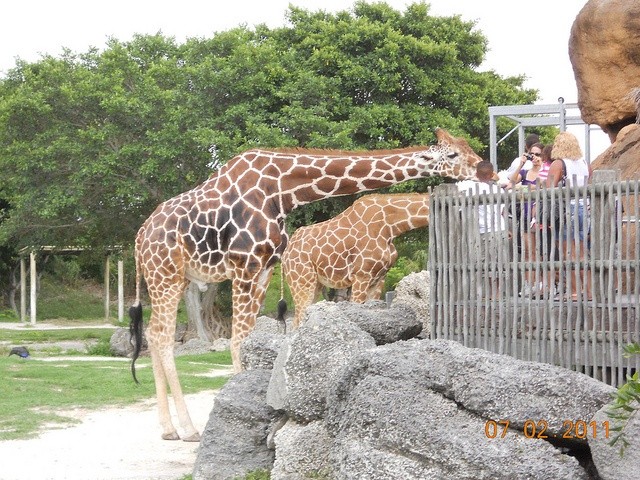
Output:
[526,155,532,160]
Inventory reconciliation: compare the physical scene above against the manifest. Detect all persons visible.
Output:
[463,161,512,301]
[538,146,566,296]
[507,135,539,181]
[546,132,593,302]
[512,142,544,298]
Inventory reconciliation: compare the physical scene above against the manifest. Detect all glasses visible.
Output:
[529,153,541,156]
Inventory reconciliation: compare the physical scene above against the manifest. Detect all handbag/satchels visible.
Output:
[534,184,568,225]
[502,201,522,220]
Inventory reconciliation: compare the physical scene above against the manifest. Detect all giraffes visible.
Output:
[126,125,500,442]
[275,192,430,334]
